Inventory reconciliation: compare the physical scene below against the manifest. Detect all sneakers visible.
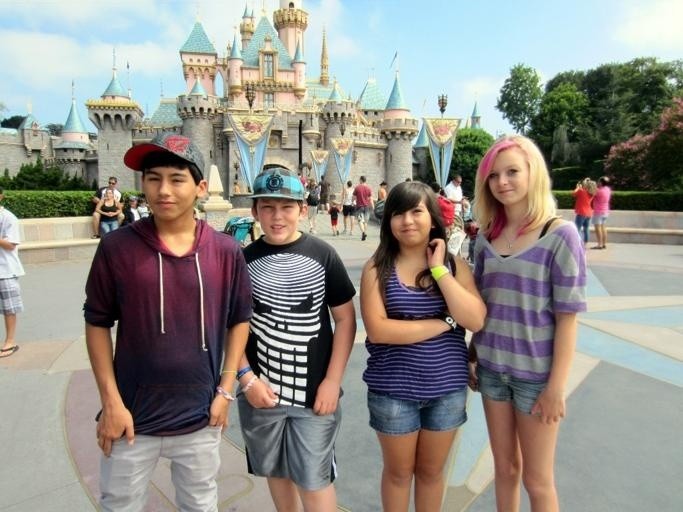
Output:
[307,227,368,242]
[589,242,607,251]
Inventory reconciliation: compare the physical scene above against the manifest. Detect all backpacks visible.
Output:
[330,206,338,220]
[307,188,319,206]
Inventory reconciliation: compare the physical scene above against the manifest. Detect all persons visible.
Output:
[464,135,588,511]
[80,129,254,511]
[589,174,612,251]
[570,177,595,244]
[0,182,26,360]
[234,167,355,511]
[359,179,488,511]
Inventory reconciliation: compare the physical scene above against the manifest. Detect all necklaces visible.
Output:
[500,217,526,250]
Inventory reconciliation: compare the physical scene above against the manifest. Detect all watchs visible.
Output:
[437,312,456,333]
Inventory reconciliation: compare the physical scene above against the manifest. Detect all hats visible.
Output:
[246,166,304,201]
[122,126,206,179]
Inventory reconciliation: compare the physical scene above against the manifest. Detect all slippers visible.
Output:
[0,338,20,359]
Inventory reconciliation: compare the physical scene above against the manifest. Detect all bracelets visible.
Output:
[240,375,258,393]
[215,385,236,403]
[235,366,252,382]
[221,369,237,375]
[429,264,449,281]
